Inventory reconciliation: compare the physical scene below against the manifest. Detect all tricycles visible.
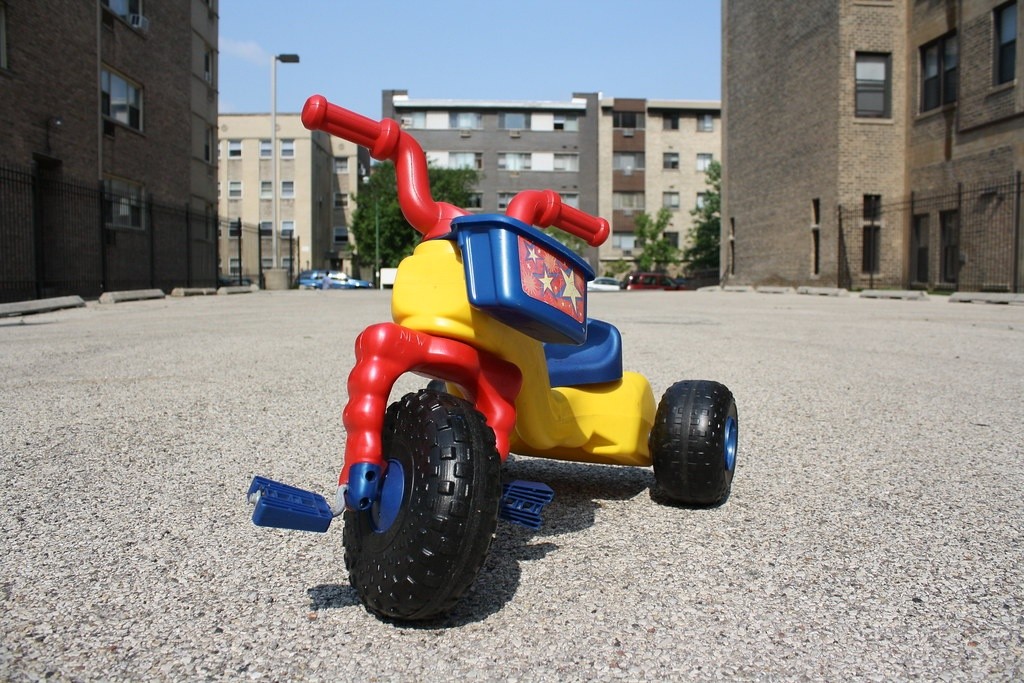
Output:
[244,93,739,624]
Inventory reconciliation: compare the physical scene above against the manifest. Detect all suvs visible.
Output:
[295,269,372,290]
[587,276,621,292]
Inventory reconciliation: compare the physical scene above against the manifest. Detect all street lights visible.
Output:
[271,53,301,271]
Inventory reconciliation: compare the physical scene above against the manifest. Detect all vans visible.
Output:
[625,272,687,291]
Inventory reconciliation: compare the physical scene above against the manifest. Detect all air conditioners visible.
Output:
[510,128,522,138]
[404,118,413,124]
[129,14,149,34]
[459,128,472,137]
